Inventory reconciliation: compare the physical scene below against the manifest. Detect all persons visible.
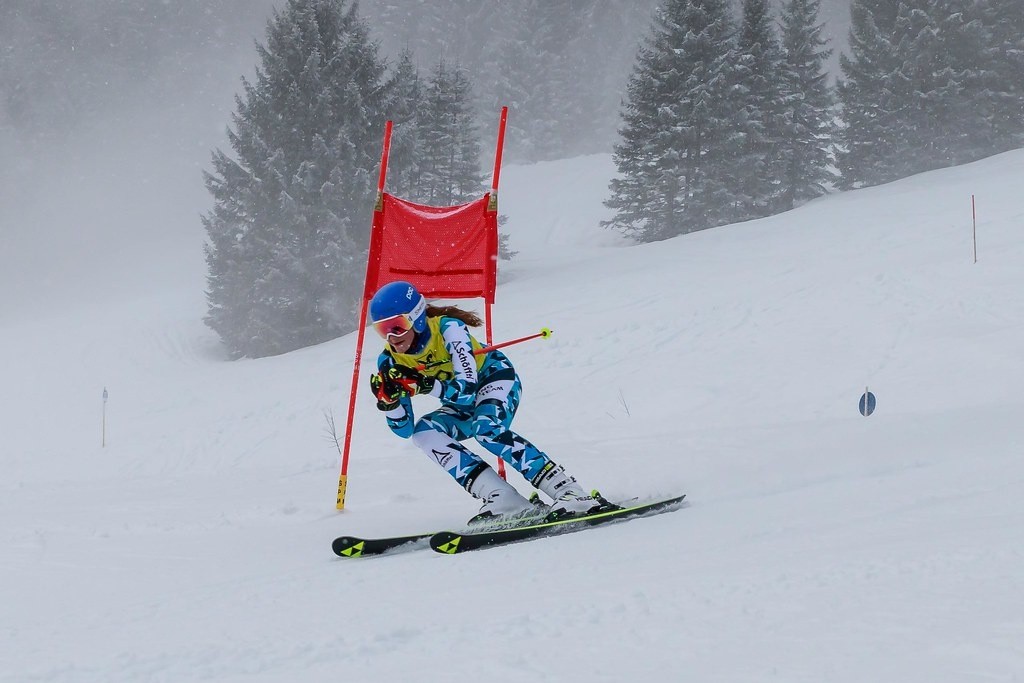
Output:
[368,280,602,526]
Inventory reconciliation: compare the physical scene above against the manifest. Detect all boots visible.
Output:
[463,461,541,527]
[532,459,602,523]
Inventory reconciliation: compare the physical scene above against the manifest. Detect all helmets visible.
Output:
[370,281,426,334]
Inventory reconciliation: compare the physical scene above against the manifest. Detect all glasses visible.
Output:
[372,314,414,341]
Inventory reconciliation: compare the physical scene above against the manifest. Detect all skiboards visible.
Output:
[330,491,687,559]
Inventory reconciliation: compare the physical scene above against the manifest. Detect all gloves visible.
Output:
[384,364,435,399]
[369,361,400,411]
